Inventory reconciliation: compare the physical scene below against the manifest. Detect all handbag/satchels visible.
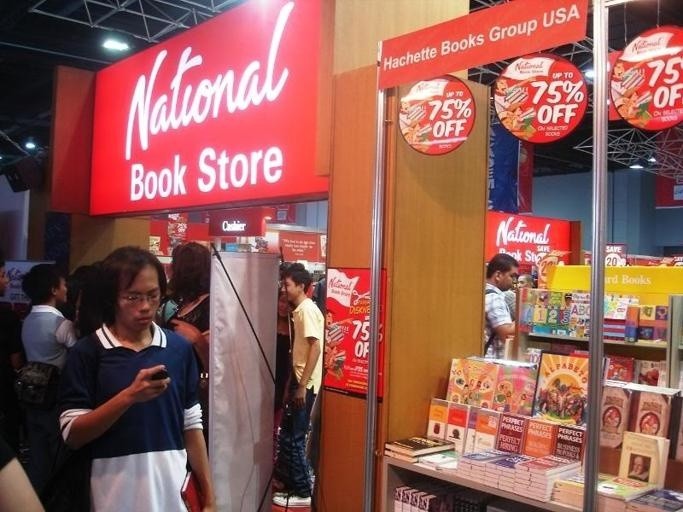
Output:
[12,361,58,411]
[35,422,85,512]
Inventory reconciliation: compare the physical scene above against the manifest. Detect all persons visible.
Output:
[483,254,538,362]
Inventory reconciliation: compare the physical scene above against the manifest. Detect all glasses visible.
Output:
[118,293,165,306]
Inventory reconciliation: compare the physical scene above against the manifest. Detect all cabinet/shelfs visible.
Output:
[376,257,682,512]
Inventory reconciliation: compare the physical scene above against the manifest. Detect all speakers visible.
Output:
[2,155,45,192]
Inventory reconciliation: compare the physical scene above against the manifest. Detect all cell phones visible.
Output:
[150,370,167,381]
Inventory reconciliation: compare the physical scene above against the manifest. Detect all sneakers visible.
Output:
[272,490,314,508]
